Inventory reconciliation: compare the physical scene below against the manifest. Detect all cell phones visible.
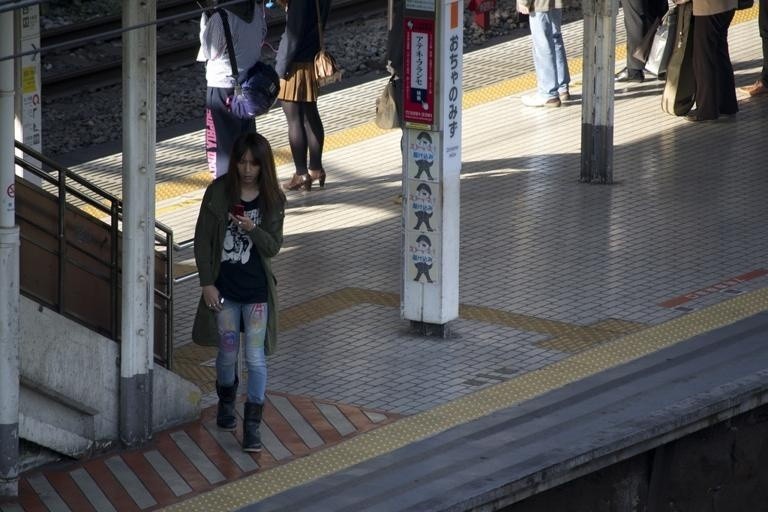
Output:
[232,204,244,218]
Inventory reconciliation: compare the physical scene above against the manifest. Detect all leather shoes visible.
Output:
[613,68,645,84]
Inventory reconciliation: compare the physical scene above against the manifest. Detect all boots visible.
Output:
[241,400,264,453]
[215,373,240,429]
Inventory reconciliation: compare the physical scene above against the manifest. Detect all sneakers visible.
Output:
[519,91,562,109]
[684,107,716,122]
[737,81,768,97]
[559,88,571,101]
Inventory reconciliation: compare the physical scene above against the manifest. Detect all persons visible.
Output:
[515,0,571,108]
[614,0,768,124]
[195,132,287,450]
[387,0,403,205]
[196,0,325,192]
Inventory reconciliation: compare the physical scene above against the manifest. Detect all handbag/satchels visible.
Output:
[230,59,280,119]
[629,6,674,67]
[643,11,677,75]
[374,74,400,132]
[313,46,340,81]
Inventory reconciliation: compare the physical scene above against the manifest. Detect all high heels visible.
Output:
[282,174,312,192]
[307,165,326,186]
